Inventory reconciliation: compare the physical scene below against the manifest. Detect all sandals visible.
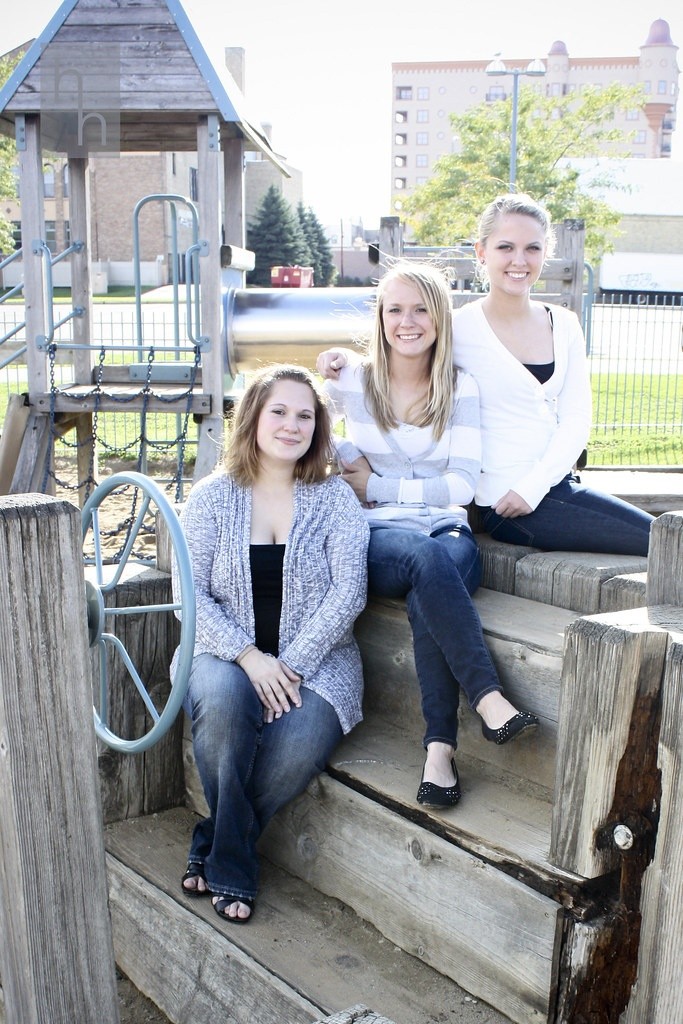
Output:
[210,892,254,922]
[182,863,210,897]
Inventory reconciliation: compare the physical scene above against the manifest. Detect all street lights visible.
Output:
[482,69,548,189]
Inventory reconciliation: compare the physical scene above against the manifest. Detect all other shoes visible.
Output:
[482,712,541,746]
[417,757,462,808]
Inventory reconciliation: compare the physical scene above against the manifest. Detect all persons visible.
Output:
[314,192,658,558]
[318,262,539,810]
[168,367,370,924]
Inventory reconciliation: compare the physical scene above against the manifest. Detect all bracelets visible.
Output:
[238,647,257,664]
